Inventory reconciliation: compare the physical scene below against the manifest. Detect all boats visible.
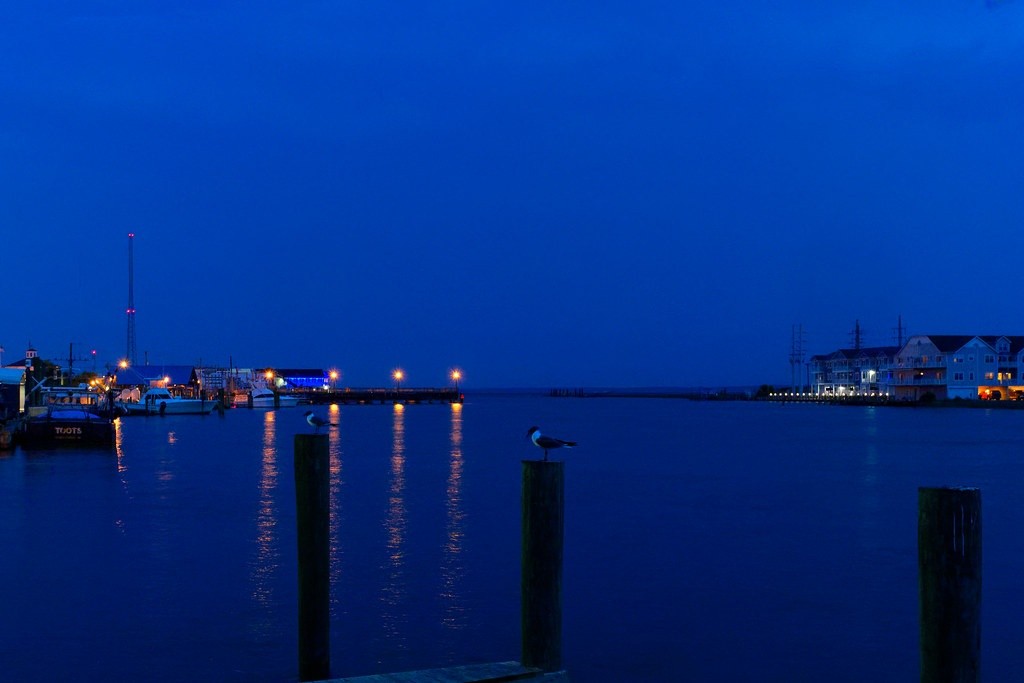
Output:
[118,386,220,420]
[250,378,297,409]
[17,376,126,447]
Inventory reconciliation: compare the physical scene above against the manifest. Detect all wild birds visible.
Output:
[303,410,340,433]
[525,426,578,461]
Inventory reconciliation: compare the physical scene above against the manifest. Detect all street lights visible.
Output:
[264,371,274,389]
[393,369,404,391]
[452,370,461,390]
[329,369,339,392]
